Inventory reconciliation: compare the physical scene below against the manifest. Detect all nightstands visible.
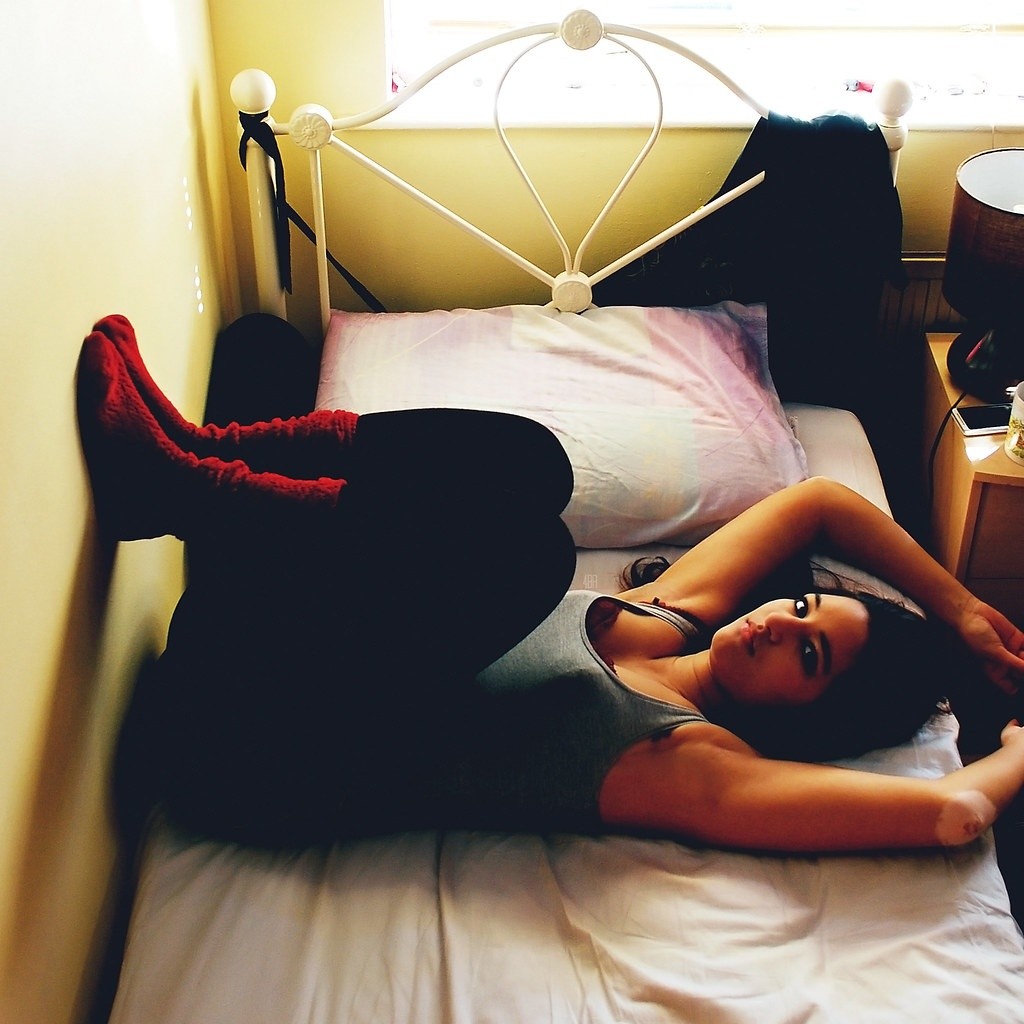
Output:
[908,328,1022,628]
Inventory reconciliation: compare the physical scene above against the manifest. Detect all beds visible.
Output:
[96,10,1023,1023]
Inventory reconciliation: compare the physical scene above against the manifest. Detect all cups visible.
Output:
[1005,381,1024,465]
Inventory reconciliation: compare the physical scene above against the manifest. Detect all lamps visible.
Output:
[945,146,1024,394]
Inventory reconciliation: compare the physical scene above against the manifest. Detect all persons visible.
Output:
[76,313,1024,849]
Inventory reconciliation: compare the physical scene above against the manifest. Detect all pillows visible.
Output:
[307,301,811,555]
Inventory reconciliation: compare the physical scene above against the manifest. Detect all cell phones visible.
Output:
[952,401,1012,437]
